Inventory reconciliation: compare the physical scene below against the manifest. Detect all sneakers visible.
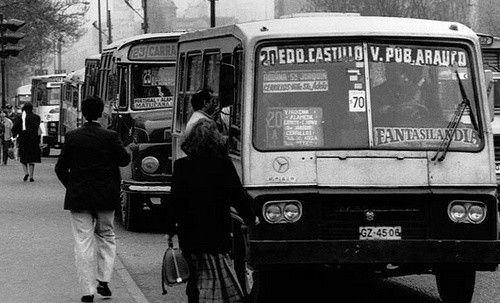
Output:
[82,295,94,303]
[97,284,112,298]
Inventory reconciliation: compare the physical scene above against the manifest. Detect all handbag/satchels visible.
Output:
[161,236,190,294]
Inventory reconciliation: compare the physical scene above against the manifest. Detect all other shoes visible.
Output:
[24,174,29,181]
[30,178,34,182]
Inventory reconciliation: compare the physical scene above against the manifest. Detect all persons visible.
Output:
[182,91,229,157]
[377,66,425,121]
[165,117,256,303]
[54,94,131,303]
[0,102,41,182]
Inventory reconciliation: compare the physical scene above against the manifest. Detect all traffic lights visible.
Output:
[3,18,26,58]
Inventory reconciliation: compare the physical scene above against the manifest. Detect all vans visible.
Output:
[14,53,100,158]
[438,69,500,214]
[93,30,185,234]
[171,13,500,303]
